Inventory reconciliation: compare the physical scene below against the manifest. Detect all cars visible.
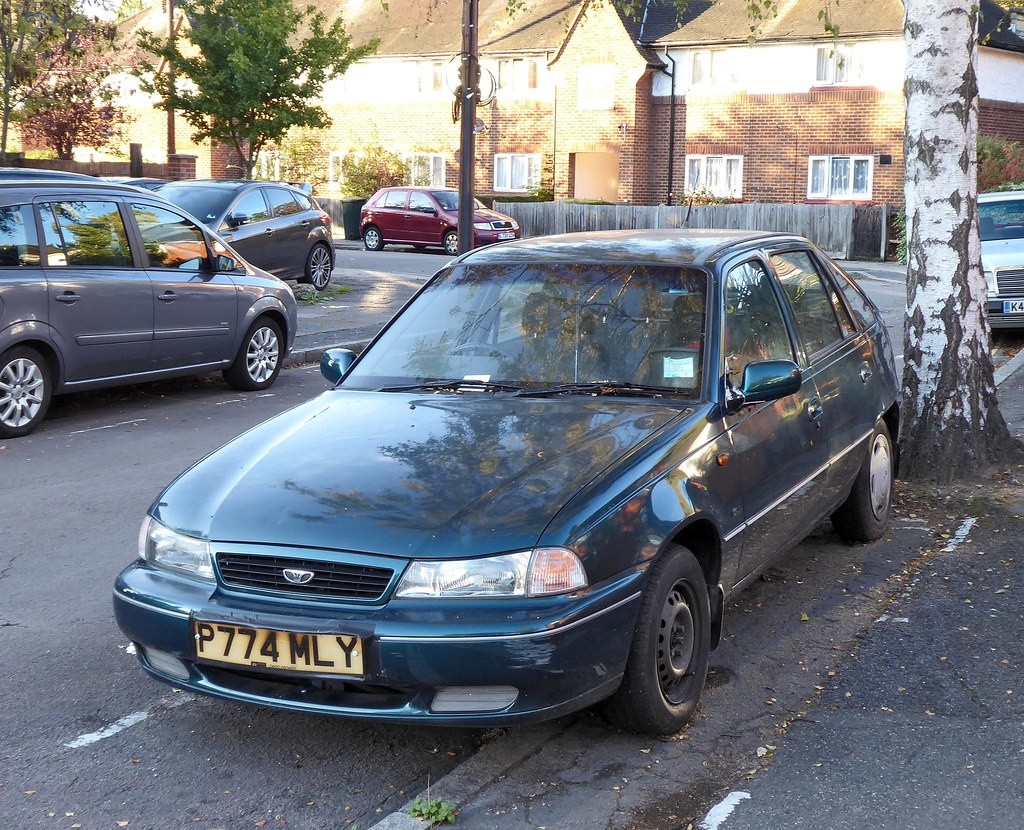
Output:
[977,190,1024,340]
[358,184,520,257]
[149,177,336,292]
[0,177,298,442]
[111,227,902,739]
